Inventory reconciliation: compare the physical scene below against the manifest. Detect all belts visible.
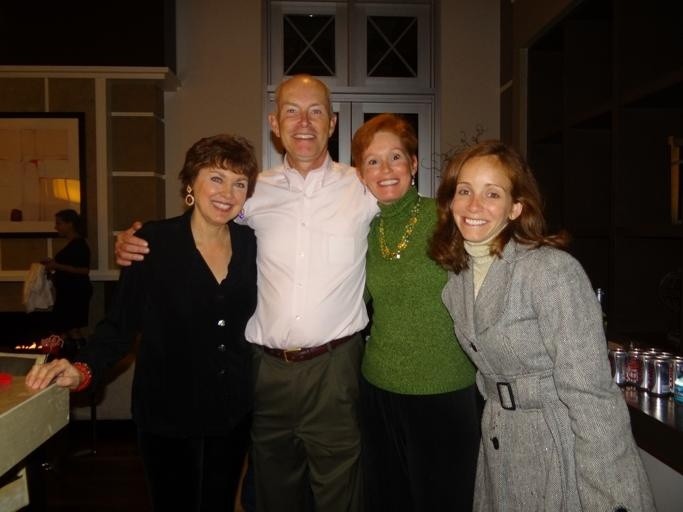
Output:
[262,331,353,361]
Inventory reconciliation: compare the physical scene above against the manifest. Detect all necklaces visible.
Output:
[379,196,422,261]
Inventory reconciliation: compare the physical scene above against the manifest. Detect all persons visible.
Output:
[431,141,656,512]
[352,115,484,511]
[41,210,89,334]
[117,76,381,512]
[26,133,260,512]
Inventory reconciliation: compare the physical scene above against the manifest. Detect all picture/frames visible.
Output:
[0,111,85,240]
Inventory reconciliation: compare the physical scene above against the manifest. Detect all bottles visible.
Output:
[597,288,609,349]
[624,347,683,402]
[608,347,628,384]
